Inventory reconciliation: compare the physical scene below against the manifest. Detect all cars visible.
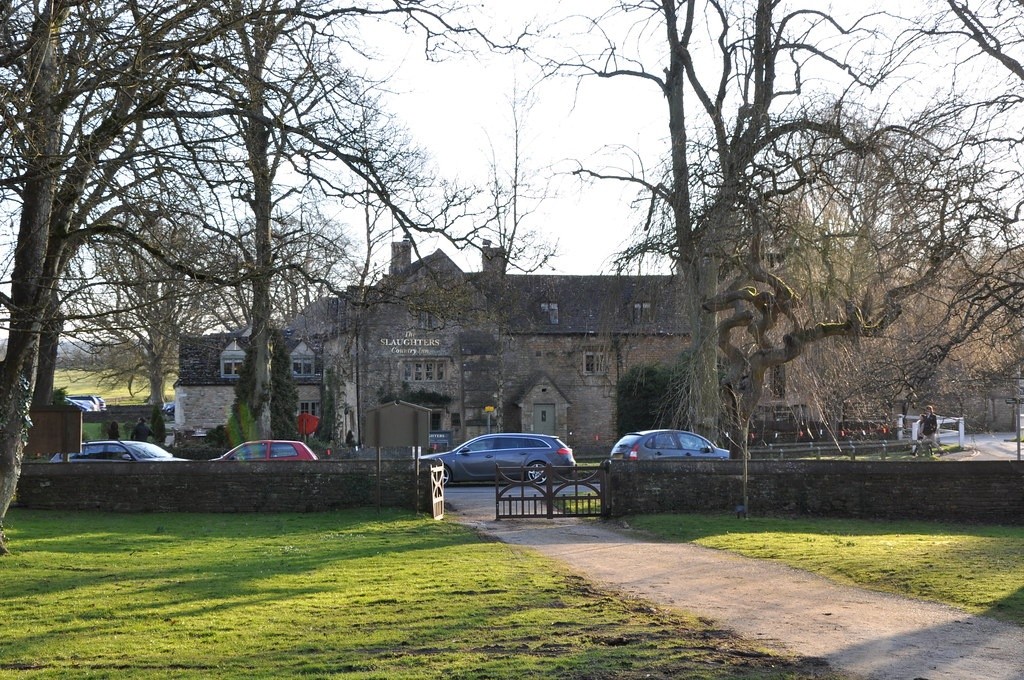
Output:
[207,440,318,461]
[163,402,175,415]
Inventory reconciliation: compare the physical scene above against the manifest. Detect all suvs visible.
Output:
[64,394,107,413]
[609,429,730,461]
[50,438,191,461]
[420,433,576,489]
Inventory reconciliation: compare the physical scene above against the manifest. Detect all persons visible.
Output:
[131,417,152,442]
[923,406,944,455]
[910,414,934,456]
[108,421,120,440]
[346,429,354,445]
